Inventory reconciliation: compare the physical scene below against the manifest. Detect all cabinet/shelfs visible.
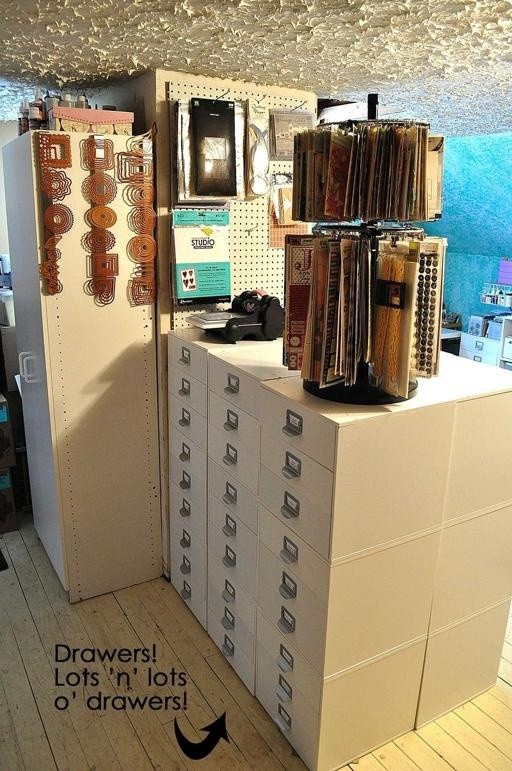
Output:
[2,129,162,604]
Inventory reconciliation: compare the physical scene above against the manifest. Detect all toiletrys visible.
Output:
[481,285,512,307]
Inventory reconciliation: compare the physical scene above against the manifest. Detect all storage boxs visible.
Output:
[0,289,19,534]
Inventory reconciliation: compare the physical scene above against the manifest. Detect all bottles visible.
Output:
[16,85,90,136]
[480,287,512,306]
